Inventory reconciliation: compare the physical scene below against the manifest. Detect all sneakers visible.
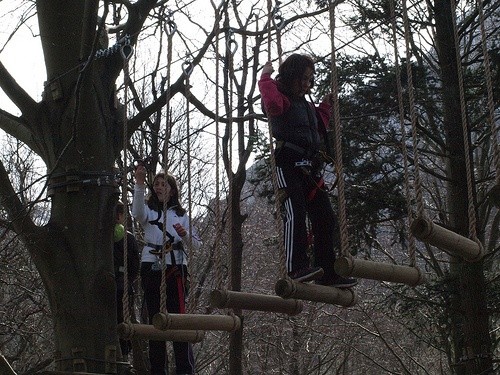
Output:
[315,268,358,287]
[289,266,325,282]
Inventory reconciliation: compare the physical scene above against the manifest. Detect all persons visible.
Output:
[258,53,357,287]
[113,166,195,375]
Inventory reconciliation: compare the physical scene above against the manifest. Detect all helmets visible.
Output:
[113,223,126,242]
